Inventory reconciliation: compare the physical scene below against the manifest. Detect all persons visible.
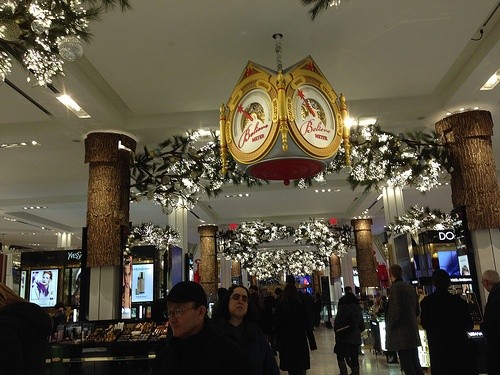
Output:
[420,269,474,375]
[34,271,54,300]
[386,264,425,375]
[480,270,500,375]
[333,286,365,375]
[212,275,332,375]
[52,302,67,340]
[151,281,257,375]
[0,283,55,375]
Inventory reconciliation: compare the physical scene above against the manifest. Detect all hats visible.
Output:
[159,280,209,309]
